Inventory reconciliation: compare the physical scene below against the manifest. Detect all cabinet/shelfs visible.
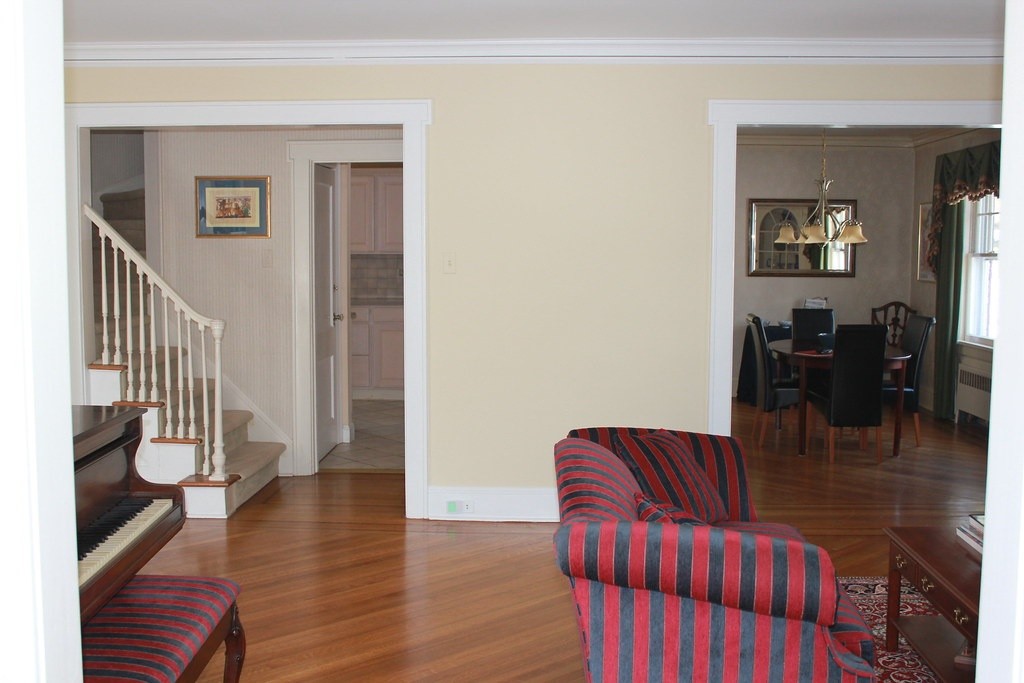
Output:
[349,168,403,255]
[351,305,403,389]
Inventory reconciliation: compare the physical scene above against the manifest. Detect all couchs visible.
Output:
[552,427,875,683]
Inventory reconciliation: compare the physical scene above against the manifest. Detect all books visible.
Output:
[955,514,984,555]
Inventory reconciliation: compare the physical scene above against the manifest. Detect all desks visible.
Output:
[736,319,792,412]
[769,339,912,458]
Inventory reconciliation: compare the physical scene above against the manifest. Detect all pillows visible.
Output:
[634,492,708,526]
[613,428,729,524]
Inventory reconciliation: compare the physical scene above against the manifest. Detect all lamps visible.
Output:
[773,207,831,244]
[801,128,868,250]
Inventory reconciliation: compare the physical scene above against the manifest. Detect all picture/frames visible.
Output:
[916,202,938,284]
[195,176,270,239]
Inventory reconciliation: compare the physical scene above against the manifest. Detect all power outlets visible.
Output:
[399,269,403,276]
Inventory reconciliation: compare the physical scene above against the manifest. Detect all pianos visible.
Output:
[71,401,190,628]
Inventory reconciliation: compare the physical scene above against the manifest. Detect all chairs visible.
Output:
[791,308,836,423]
[859,314,936,449]
[807,324,889,464]
[745,312,814,447]
[871,301,918,348]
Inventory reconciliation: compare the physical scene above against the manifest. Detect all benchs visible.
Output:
[81,574,247,683]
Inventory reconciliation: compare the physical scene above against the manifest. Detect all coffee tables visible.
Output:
[883,526,984,683]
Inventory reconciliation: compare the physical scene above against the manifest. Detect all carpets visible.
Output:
[836,577,943,683]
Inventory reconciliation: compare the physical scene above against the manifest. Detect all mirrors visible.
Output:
[748,199,857,278]
